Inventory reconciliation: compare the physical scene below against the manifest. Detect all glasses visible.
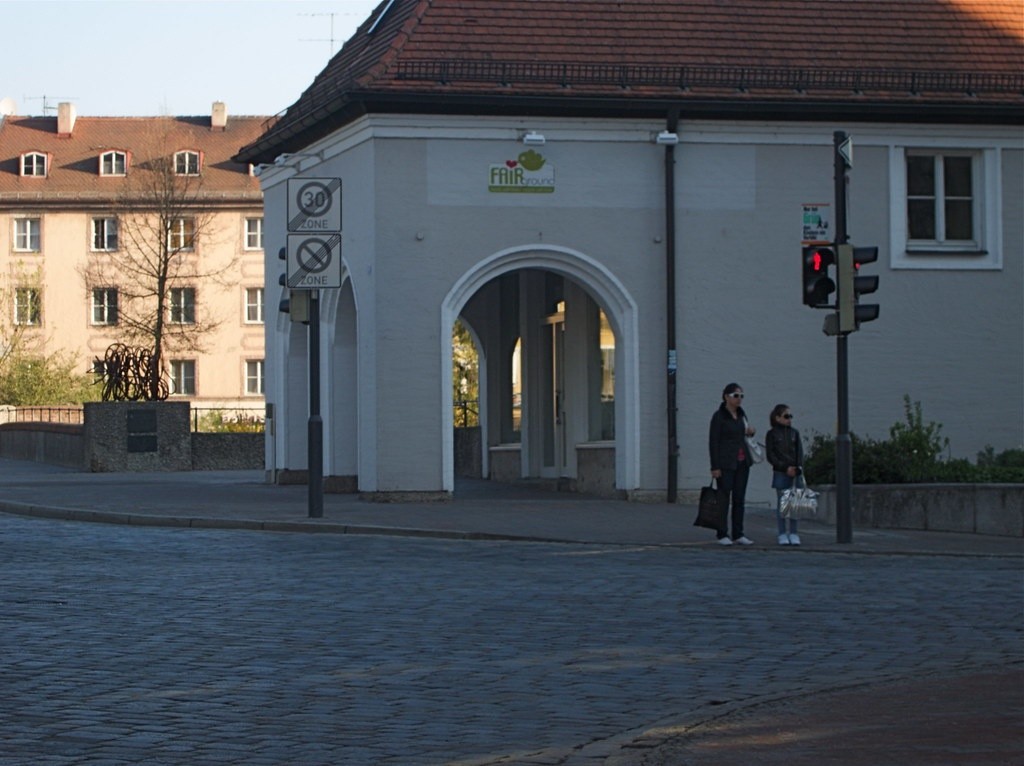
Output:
[728,392,744,399]
[780,413,792,419]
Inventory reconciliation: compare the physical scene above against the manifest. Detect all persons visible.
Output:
[709,382,756,547]
[765,401,807,546]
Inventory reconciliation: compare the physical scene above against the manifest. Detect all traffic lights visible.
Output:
[801,240,837,308]
[279,248,311,325]
[838,243,881,332]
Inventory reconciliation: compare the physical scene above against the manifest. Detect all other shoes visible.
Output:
[735,536,754,544]
[718,536,733,545]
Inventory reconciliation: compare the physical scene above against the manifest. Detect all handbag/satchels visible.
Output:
[779,467,820,520]
[742,417,764,464]
[693,476,728,530]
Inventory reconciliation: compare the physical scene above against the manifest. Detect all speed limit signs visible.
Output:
[284,177,347,237]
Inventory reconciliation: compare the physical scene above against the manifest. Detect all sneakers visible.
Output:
[777,534,789,545]
[789,533,801,545]
